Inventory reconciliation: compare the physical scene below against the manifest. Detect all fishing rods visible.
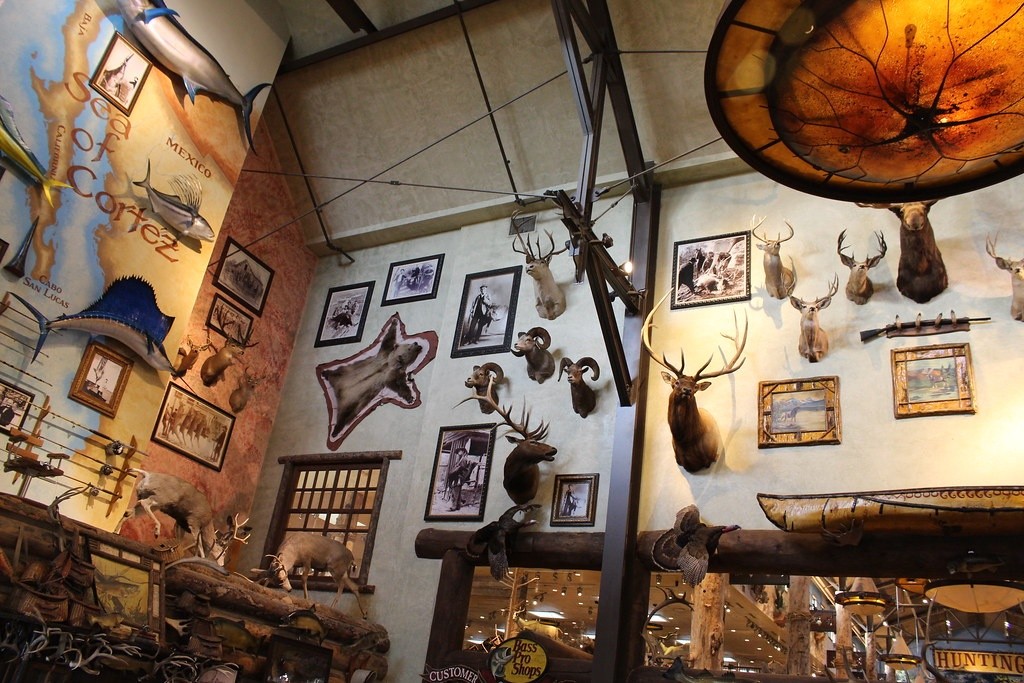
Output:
[0,394,151,500]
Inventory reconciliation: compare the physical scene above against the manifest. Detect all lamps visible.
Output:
[877,587,922,670]
[833,578,890,616]
[656,574,662,585]
[561,587,567,596]
[577,587,583,596]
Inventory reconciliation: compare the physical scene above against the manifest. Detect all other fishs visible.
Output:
[131,157,215,246]
[0,92,75,210]
[117,0,276,159]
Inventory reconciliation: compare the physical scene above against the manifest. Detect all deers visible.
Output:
[640,211,890,475]
[984,237,1024,323]
[509,207,573,321]
[452,372,559,506]
[178,303,268,414]
[0,438,368,620]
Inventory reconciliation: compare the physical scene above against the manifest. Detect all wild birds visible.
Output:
[650,503,743,590]
[464,503,544,582]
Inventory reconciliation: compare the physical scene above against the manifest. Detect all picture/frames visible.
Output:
[549,473,600,527]
[379,252,446,307]
[314,280,375,346]
[890,342,975,419]
[204,293,255,347]
[450,264,524,358]
[0,378,36,436]
[669,229,752,310]
[423,422,497,523]
[757,374,842,448]
[89,31,152,116]
[212,237,275,319]
[151,380,237,474]
[68,341,134,418]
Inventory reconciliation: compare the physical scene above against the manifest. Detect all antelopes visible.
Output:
[558,356,601,419]
[507,326,556,385]
[462,361,504,416]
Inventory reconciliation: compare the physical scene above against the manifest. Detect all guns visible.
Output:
[859,316,992,343]
